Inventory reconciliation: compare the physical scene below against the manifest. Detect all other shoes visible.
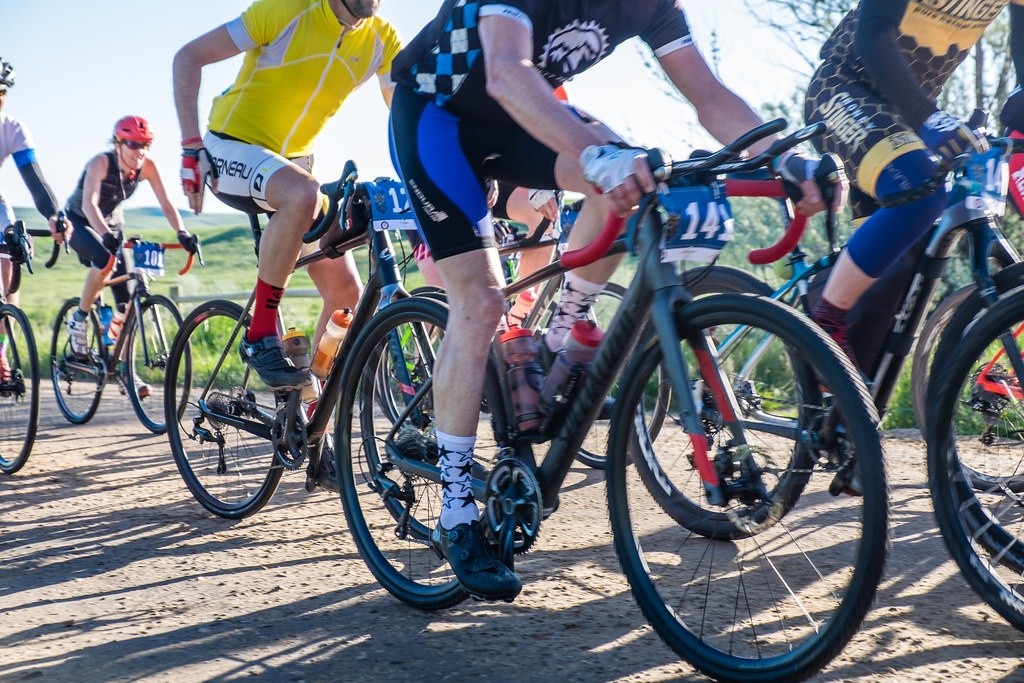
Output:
[0,358,11,383]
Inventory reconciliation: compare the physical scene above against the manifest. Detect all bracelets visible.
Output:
[181,136,203,145]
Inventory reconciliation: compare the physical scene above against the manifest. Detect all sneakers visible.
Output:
[239,330,307,386]
[536,336,616,419]
[822,434,866,496]
[120,374,149,396]
[67,313,88,360]
[430,516,522,601]
[808,312,858,372]
[306,434,341,492]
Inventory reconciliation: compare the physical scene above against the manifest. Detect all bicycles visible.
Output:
[0,210,72,474]
[376,105,1024,635]
[333,119,893,683]
[162,159,511,552]
[48,220,205,434]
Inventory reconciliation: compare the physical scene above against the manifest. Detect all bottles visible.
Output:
[507,287,538,326]
[310,308,353,379]
[500,326,544,432]
[544,320,602,405]
[108,303,130,339]
[282,327,322,404]
[98,304,114,346]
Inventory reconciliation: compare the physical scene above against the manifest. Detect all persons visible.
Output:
[808,0,1024,376]
[173,0,402,492]
[0,57,74,384]
[388,0,850,602]
[413,85,569,351]
[63,114,196,397]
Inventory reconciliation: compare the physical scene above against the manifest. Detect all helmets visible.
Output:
[0,55,16,88]
[113,115,152,144]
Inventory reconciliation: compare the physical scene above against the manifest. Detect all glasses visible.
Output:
[0,87,8,98]
[121,140,149,149]
